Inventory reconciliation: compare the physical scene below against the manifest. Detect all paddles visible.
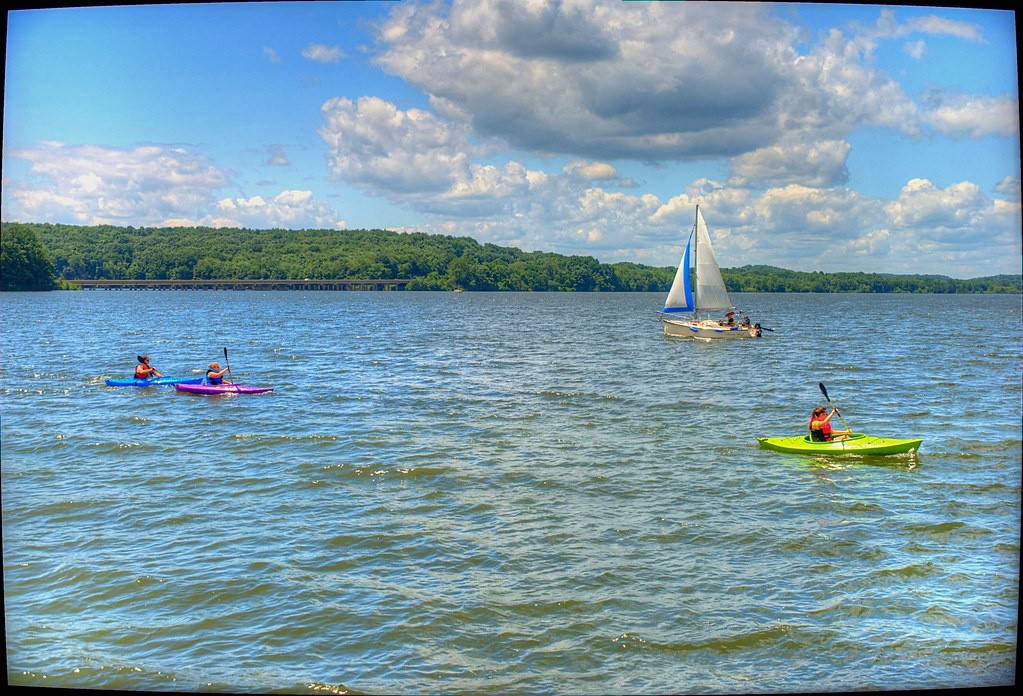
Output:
[136,355,165,375]
[222,346,234,384]
[818,381,851,432]
[759,326,775,333]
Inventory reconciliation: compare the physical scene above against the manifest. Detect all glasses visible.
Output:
[218,366,219,368]
[147,360,149,361]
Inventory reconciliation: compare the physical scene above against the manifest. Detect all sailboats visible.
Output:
[659,206,763,339]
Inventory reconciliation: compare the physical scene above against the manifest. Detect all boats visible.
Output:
[174,381,274,395]
[757,429,924,458]
[105,375,206,387]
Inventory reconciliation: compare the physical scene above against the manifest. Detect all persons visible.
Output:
[206,362,231,385]
[756,324,762,337]
[742,316,750,326]
[728,315,734,325]
[134,357,163,378]
[809,407,852,442]
